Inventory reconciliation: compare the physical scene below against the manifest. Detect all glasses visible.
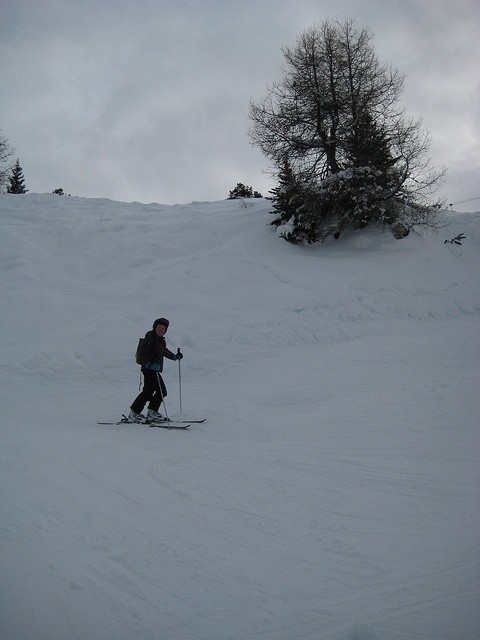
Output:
[157,318,169,326]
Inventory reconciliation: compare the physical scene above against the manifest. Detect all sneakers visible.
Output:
[128,411,146,423]
[146,409,164,422]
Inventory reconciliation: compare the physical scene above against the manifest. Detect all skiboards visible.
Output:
[98,418,207,429]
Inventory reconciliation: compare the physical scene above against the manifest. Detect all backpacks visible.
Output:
[135,335,155,365]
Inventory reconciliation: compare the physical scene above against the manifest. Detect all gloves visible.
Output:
[175,352,183,361]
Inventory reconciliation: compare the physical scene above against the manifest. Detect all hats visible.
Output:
[153,317,169,335]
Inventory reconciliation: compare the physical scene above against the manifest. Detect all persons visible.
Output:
[127,317,183,423]
